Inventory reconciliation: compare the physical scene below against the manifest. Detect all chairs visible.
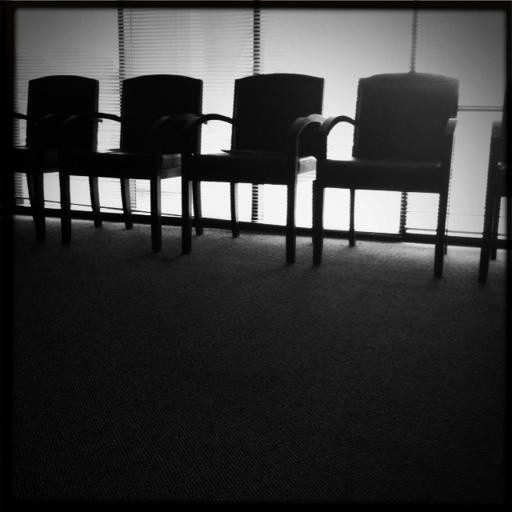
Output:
[94,73,205,252]
[8,74,101,229]
[301,70,461,277]
[477,120,511,283]
[182,73,327,264]
